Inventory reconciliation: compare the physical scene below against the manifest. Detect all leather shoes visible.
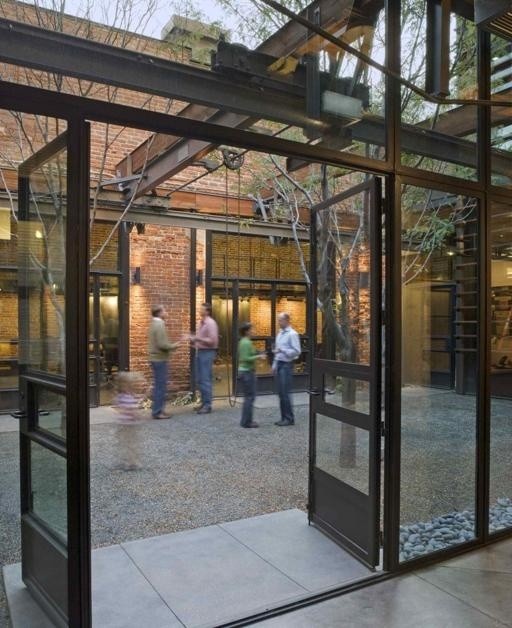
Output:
[152,403,294,428]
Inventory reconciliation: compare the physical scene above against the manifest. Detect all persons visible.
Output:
[185,303,218,414]
[238,322,267,428]
[146,303,183,418]
[268,313,302,427]
